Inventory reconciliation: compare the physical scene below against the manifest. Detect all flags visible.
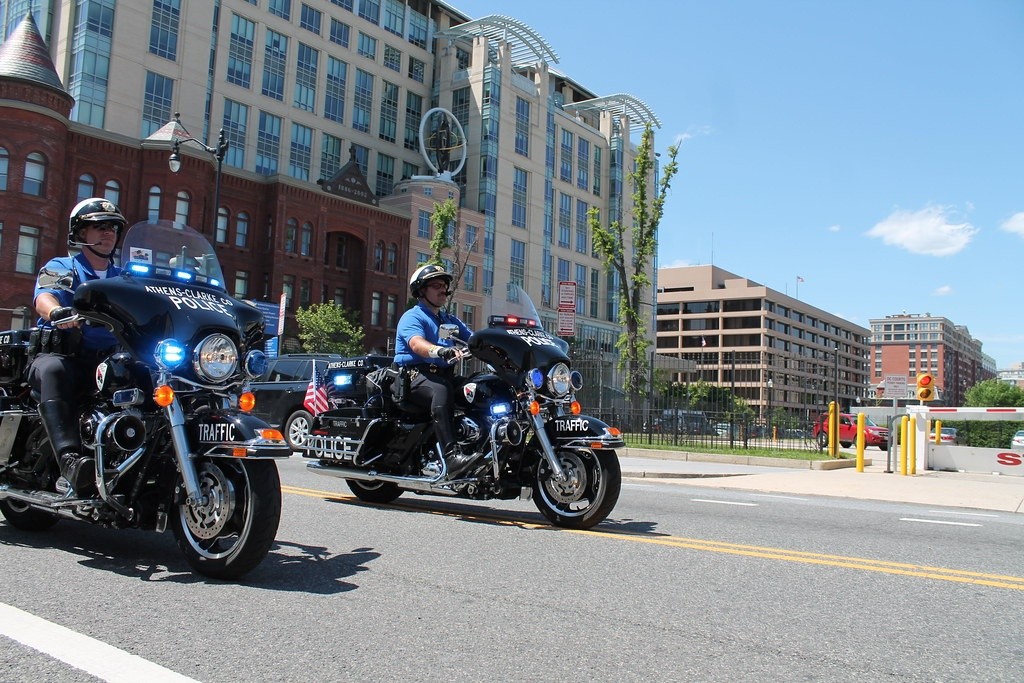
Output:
[303,365,330,416]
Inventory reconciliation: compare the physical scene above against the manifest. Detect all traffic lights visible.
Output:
[917,373,935,401]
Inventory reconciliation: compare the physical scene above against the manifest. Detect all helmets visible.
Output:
[409,265,453,299]
[69,197,128,239]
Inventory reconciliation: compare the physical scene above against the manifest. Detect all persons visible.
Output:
[24,196,126,499]
[393,265,484,482]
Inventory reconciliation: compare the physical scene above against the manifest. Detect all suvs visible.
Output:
[812,412,893,451]
[235,353,342,452]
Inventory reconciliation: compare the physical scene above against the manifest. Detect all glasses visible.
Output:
[421,283,448,290]
[90,223,119,233]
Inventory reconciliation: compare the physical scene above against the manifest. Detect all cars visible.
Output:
[928,427,960,445]
[643,413,807,439]
[1010,430,1024,450]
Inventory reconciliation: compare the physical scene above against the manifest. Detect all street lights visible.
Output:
[169,128,230,254]
[767,378,773,409]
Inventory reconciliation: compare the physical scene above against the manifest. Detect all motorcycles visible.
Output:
[299,283,626,530]
[0,217,293,583]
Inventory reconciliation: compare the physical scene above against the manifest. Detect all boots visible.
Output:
[39,399,95,498]
[431,405,484,480]
